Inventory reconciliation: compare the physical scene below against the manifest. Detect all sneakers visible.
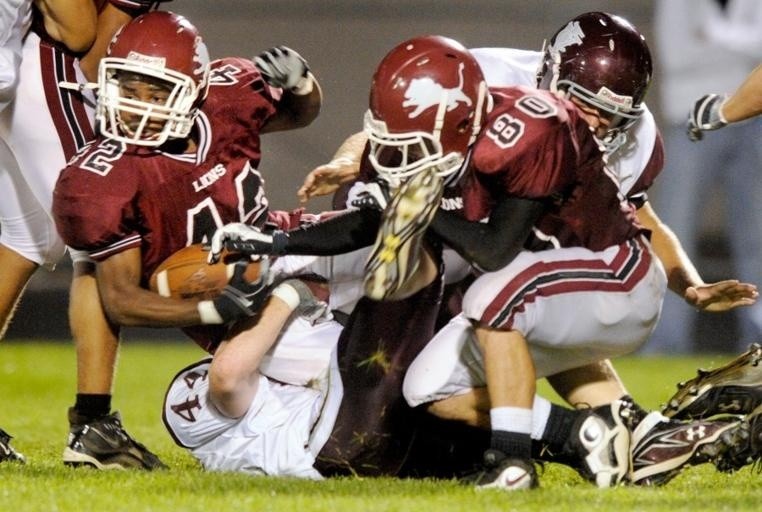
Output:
[628,410,743,487]
[561,400,634,489]
[456,449,539,491]
[0,428,25,466]
[662,342,761,422]
[63,408,170,472]
[363,167,444,303]
[715,411,762,474]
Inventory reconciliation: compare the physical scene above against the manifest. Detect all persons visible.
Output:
[1,0,471,493]
[148,10,762,491]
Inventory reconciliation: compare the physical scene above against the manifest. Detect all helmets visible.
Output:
[363,36,494,189]
[94,11,210,148]
[535,12,652,164]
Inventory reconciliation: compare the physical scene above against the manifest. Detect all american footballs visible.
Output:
[148,244,262,301]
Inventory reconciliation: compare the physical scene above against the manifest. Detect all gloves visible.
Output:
[253,45,314,96]
[197,254,282,325]
[272,272,329,311]
[351,178,391,212]
[685,93,729,142]
[207,221,283,265]
